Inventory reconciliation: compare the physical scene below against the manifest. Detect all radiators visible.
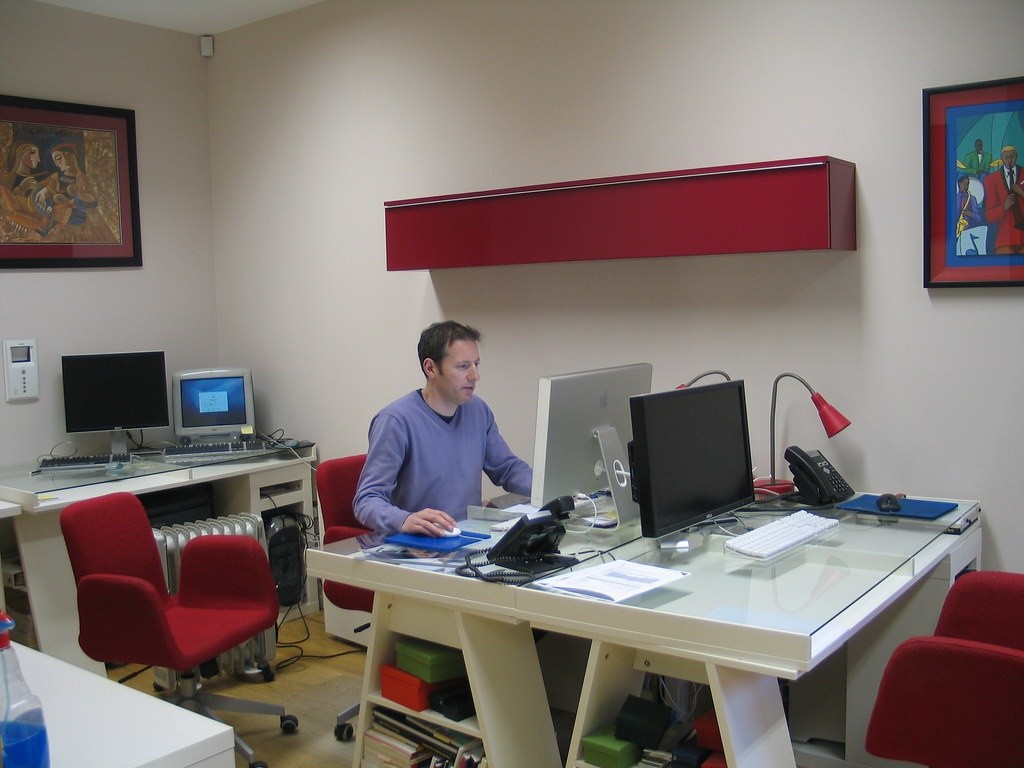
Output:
[149,512,278,692]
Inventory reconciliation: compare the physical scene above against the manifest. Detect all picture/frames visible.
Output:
[923,76,1024,288]
[0,96,144,268]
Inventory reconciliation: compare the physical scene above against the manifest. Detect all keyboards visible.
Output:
[488,494,605,532]
[37,453,132,470]
[724,509,839,561]
[162,440,267,458]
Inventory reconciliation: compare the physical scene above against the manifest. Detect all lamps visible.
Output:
[673,370,735,391]
[755,369,851,496]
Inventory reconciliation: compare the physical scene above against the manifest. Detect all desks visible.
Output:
[10,641,238,768]
[0,443,319,677]
[303,493,984,767]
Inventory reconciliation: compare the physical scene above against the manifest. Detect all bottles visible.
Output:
[0,612,52,768]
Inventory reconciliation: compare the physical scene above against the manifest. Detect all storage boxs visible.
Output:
[377,665,429,712]
[394,640,467,683]
[695,711,726,752]
[579,725,641,768]
[421,683,476,723]
[615,694,676,743]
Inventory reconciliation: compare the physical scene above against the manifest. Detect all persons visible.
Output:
[352,319,533,538]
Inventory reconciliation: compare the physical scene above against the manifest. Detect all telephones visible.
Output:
[784,445,856,507]
[484,495,579,573]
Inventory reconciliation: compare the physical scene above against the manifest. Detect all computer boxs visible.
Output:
[265,513,304,609]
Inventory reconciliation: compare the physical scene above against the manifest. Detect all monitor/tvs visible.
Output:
[57,349,172,454]
[530,364,652,532]
[627,379,755,548]
[172,368,256,446]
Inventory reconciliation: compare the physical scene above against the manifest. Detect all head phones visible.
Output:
[265,428,284,445]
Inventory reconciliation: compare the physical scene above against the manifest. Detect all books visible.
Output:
[360,705,488,768]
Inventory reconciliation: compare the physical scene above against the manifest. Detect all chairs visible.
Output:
[318,455,404,741]
[865,570,1024,768]
[61,490,297,768]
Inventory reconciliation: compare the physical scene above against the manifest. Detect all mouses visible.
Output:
[875,493,901,512]
[432,522,461,538]
[105,462,124,470]
[284,440,300,446]
